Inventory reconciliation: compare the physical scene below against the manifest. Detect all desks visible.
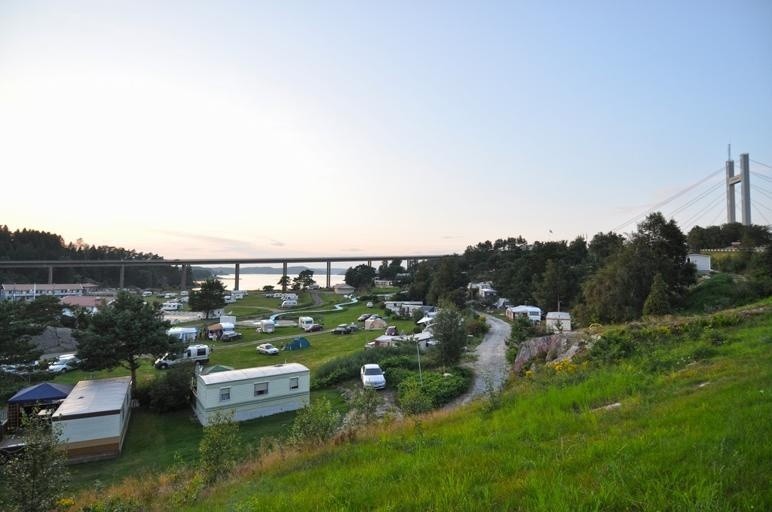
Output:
[38,409,54,415]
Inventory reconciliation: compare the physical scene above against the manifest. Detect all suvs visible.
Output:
[221,331,243,341]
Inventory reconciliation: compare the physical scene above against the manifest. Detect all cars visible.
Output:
[47,353,83,376]
[357,296,365,301]
[360,363,387,392]
[385,325,398,336]
[256,344,279,355]
[367,301,374,308]
[305,323,322,332]
[333,323,351,335]
[357,313,382,321]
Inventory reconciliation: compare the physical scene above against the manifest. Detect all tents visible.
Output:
[364,314,388,329]
[282,335,311,351]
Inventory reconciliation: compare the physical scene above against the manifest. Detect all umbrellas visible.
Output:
[417,316,434,326]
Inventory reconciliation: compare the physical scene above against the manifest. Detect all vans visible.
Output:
[155,344,211,371]
[142,288,252,312]
[265,291,298,309]
